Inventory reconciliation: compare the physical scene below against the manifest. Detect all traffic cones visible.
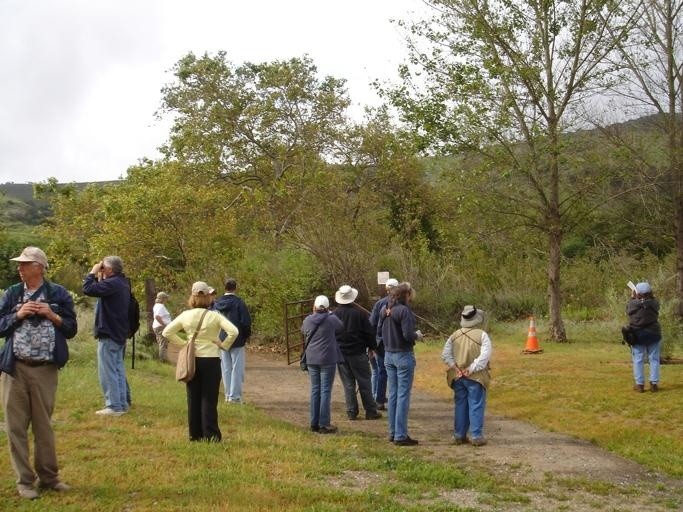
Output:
[521,316,543,354]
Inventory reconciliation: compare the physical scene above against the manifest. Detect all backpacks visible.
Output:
[370,302,395,357]
[127,274,141,339]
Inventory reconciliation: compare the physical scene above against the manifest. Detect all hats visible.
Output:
[192,281,215,296]
[460,304,485,328]
[335,285,358,304]
[157,292,170,298]
[385,278,399,289]
[9,246,48,268]
[314,295,329,309]
[635,282,650,294]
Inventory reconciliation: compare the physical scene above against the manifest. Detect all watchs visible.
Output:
[54,314,59,324]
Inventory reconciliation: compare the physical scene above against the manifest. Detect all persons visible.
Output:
[331,283,383,421]
[0,246,79,500]
[150,291,173,364]
[624,281,663,394]
[298,294,346,434]
[438,304,493,447]
[160,279,240,442]
[364,277,397,411]
[213,279,253,405]
[80,255,132,418]
[374,281,425,448]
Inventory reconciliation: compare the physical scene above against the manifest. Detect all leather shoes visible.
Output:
[472,436,487,445]
[365,412,382,420]
[347,411,361,420]
[16,482,42,500]
[455,435,470,445]
[395,437,419,446]
[38,478,73,491]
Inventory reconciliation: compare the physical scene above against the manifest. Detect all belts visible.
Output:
[15,356,55,368]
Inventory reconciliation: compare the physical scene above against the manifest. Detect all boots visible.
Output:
[649,382,658,393]
[632,384,644,393]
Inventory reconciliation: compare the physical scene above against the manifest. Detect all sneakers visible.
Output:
[310,425,321,432]
[320,425,338,434]
[94,408,125,417]
